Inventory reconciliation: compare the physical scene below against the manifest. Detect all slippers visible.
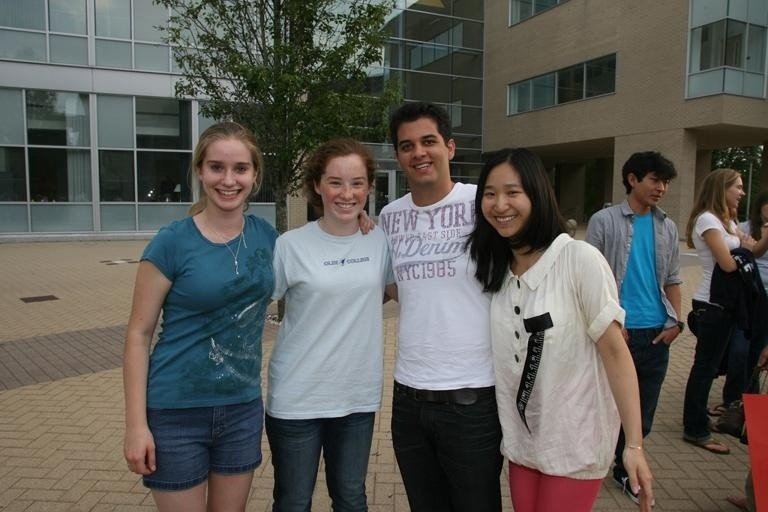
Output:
[683,436,729,454]
[711,422,720,432]
[707,405,727,415]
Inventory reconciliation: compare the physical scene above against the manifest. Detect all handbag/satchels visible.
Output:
[723,400,748,445]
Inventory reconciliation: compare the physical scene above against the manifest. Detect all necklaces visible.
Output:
[206,205,249,275]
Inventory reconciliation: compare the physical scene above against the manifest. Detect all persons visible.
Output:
[679,167,768,454]
[707,191,768,415]
[267,139,398,511]
[122,124,377,511]
[582,149,687,505]
[459,145,659,512]
[381,104,510,512]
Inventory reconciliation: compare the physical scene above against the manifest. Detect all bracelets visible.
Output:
[624,443,643,451]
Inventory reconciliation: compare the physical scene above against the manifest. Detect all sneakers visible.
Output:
[613,473,655,507]
